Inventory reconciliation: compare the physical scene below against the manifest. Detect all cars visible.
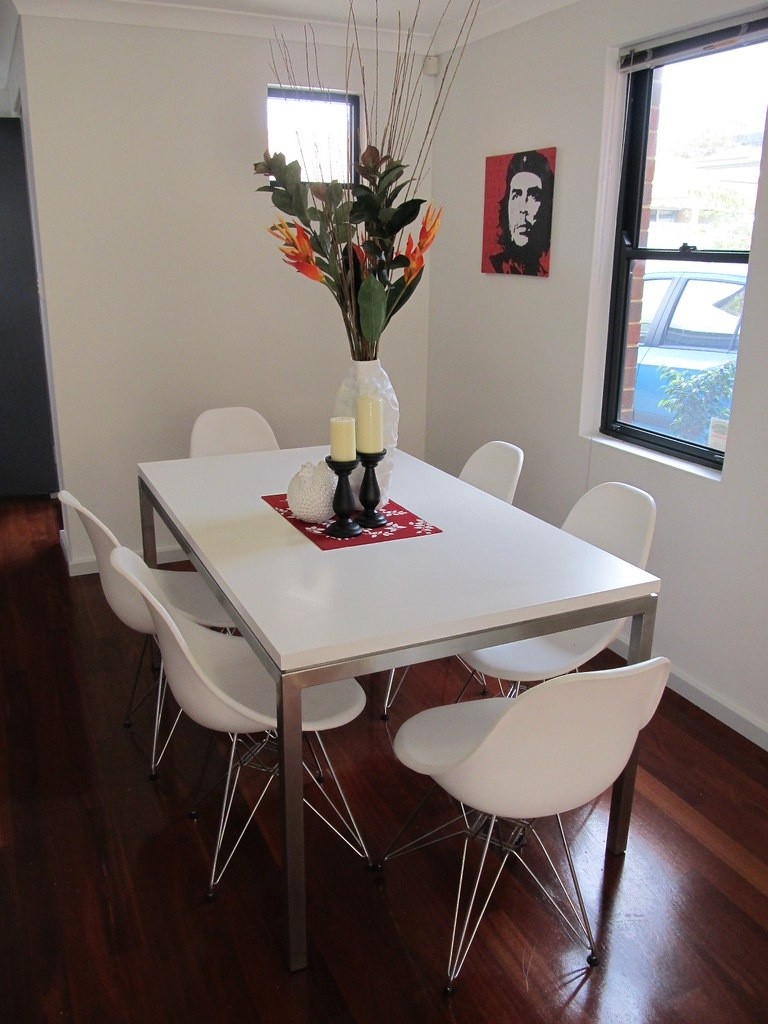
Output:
[633,271,748,446]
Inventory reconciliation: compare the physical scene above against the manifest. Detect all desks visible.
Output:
[139,440,661,976]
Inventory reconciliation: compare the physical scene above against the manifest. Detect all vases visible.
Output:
[337,359,400,512]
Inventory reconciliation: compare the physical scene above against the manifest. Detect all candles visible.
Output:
[354,397,382,451]
[329,416,357,461]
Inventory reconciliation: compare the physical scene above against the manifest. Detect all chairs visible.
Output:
[111,551,379,904]
[382,481,658,720]
[381,658,673,993]
[190,406,281,457]
[58,491,253,747]
[381,439,523,717]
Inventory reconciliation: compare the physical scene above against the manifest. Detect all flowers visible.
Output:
[252,0,482,360]
